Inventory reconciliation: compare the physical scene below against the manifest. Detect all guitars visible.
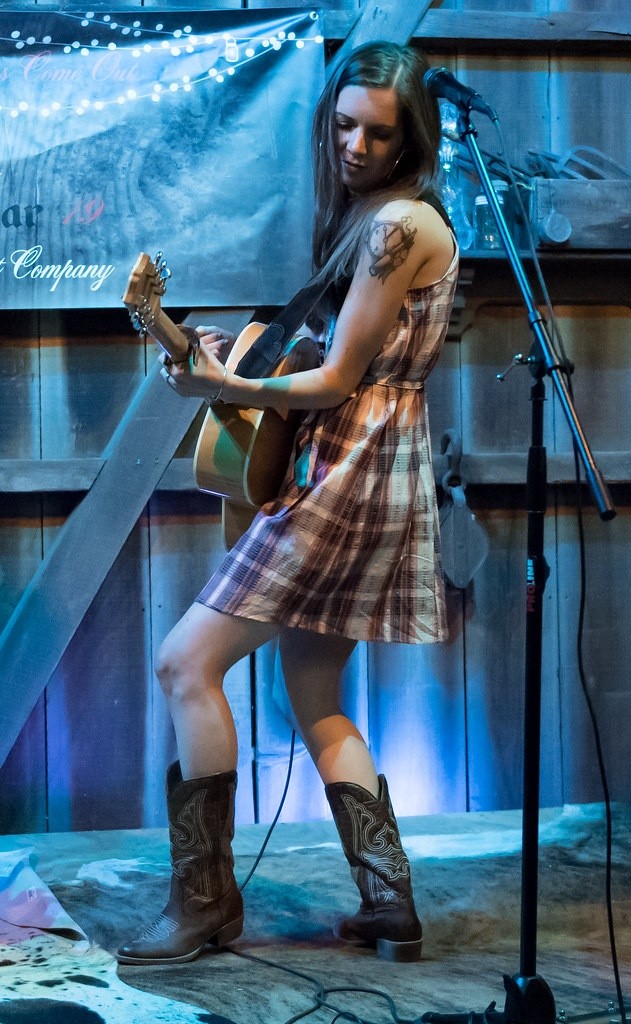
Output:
[119,250,325,558]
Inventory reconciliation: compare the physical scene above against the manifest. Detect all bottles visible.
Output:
[474,194,507,250]
[434,103,474,252]
[480,180,515,247]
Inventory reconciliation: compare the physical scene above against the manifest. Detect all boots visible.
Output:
[114,759,245,965]
[324,774,424,962]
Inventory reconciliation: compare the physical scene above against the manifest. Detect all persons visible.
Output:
[116,39,460,965]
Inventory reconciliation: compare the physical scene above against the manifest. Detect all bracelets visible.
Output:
[212,367,227,401]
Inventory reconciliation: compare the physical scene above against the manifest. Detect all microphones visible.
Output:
[422,66,497,121]
